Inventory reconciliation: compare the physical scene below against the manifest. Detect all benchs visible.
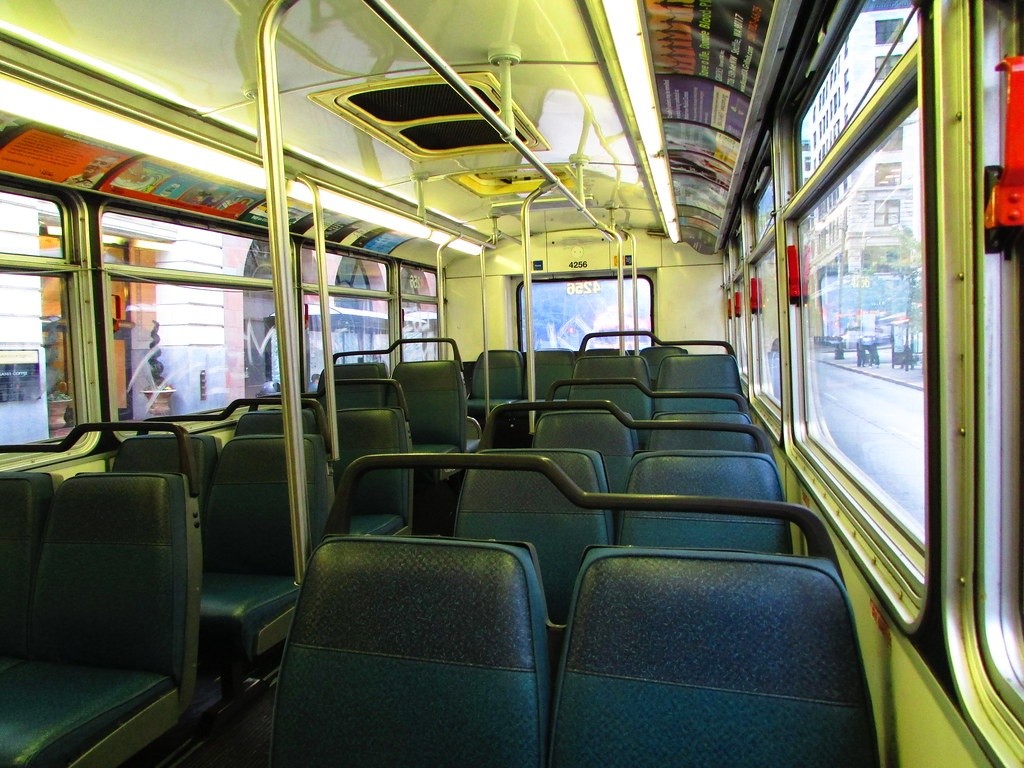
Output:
[0,329,882,767]
